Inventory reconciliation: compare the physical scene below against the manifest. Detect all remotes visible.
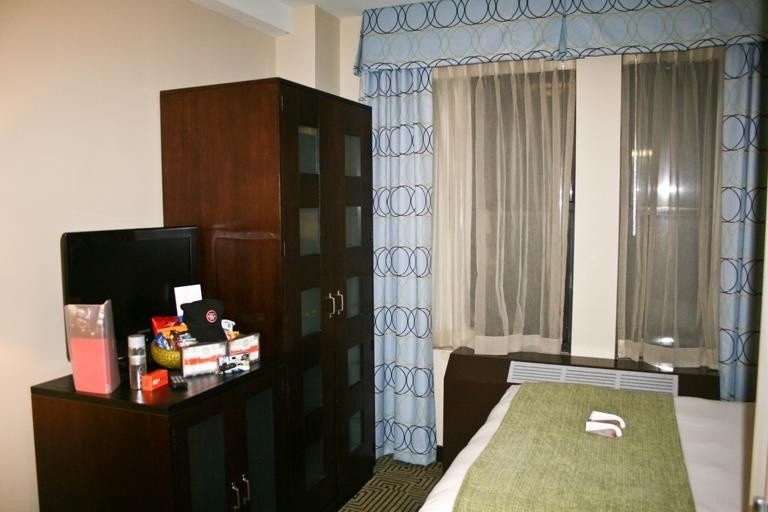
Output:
[168,367,186,389]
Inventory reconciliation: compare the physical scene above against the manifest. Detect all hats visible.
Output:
[181,299,228,342]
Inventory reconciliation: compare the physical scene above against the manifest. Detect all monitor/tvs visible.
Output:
[60,225,199,362]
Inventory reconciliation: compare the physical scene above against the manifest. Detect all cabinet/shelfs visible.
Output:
[442,346,720,472]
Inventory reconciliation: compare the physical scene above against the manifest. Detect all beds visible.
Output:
[418,380,755,512]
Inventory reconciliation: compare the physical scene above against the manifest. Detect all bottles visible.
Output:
[127,333,149,391]
